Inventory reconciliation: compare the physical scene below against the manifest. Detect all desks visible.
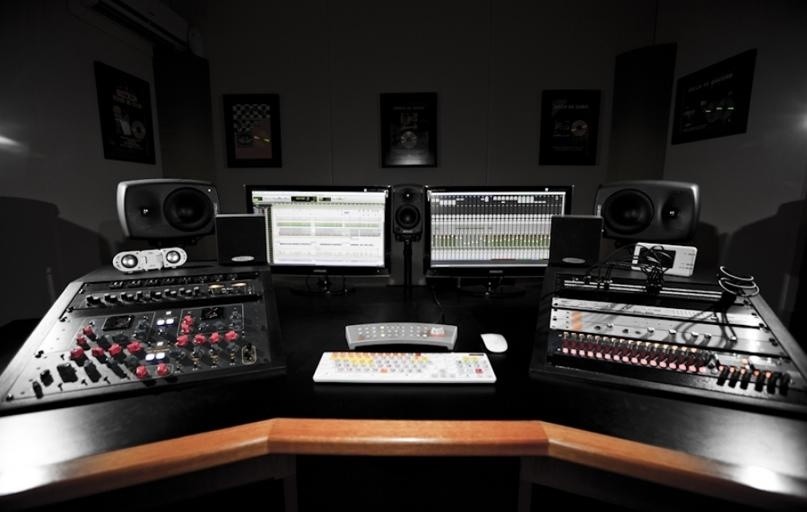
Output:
[0,266,807,512]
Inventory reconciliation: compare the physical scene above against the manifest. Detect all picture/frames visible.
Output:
[668,46,759,147]
[221,92,283,169]
[378,91,438,169]
[537,88,602,166]
[92,59,157,166]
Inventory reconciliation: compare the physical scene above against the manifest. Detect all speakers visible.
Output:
[549,215,602,269]
[112,247,188,274]
[116,180,222,237]
[392,183,426,234]
[215,214,266,266]
[593,183,700,242]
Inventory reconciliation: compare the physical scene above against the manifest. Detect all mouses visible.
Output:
[481,332,509,353]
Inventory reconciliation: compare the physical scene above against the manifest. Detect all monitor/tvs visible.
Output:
[424,186,573,277]
[245,183,390,276]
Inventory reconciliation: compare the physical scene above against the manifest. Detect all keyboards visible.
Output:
[313,350,497,384]
